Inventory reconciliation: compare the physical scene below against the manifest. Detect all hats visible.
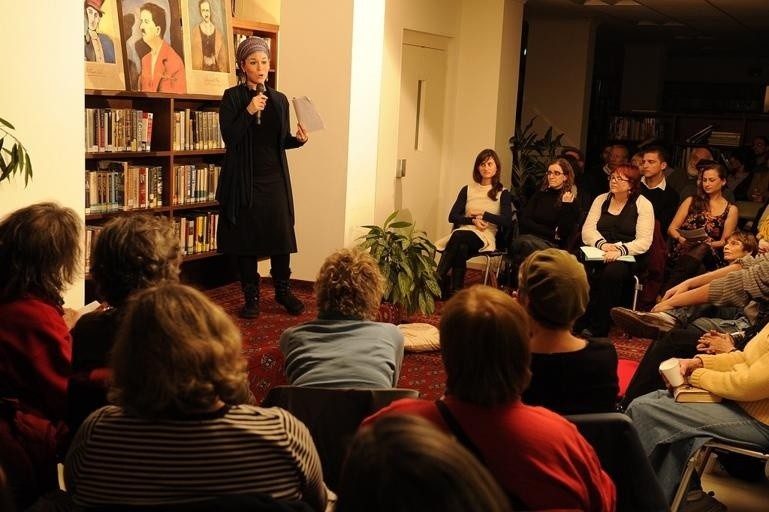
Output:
[523,247,591,324]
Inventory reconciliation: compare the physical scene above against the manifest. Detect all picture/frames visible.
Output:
[180,0,239,96]
[83,0,133,90]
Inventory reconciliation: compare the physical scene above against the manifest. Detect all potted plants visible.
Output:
[351,205,447,327]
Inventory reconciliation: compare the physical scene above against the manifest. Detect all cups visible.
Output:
[659,358,684,388]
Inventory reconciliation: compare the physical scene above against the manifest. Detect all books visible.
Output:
[614,118,742,173]
[83,99,226,277]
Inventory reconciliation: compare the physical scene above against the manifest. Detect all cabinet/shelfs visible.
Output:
[672,115,744,172]
[231,17,280,92]
[175,88,244,292]
[83,88,174,301]
[590,110,672,160]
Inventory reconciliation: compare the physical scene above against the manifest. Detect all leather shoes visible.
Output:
[611,306,679,342]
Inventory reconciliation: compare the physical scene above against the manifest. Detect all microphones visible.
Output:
[256,83,264,124]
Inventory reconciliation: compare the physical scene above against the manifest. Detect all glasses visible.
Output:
[608,175,629,183]
[544,171,566,177]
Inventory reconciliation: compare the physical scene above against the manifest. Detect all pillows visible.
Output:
[397,321,441,353]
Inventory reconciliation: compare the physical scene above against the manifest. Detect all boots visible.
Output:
[269,269,305,314]
[240,274,261,319]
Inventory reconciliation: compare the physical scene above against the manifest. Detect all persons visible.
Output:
[441,140,738,339]
[61,215,185,439]
[214,36,310,318]
[512,206,769,511]
[84,0,114,62]
[190,0,227,73]
[60,285,337,511]
[339,280,617,511]
[0,198,76,512]
[133,3,188,94]
[280,247,409,390]
[333,413,512,512]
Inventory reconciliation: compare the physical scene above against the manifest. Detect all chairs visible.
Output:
[623,268,643,340]
[558,412,670,511]
[255,385,419,483]
[463,252,505,288]
[666,423,769,509]
